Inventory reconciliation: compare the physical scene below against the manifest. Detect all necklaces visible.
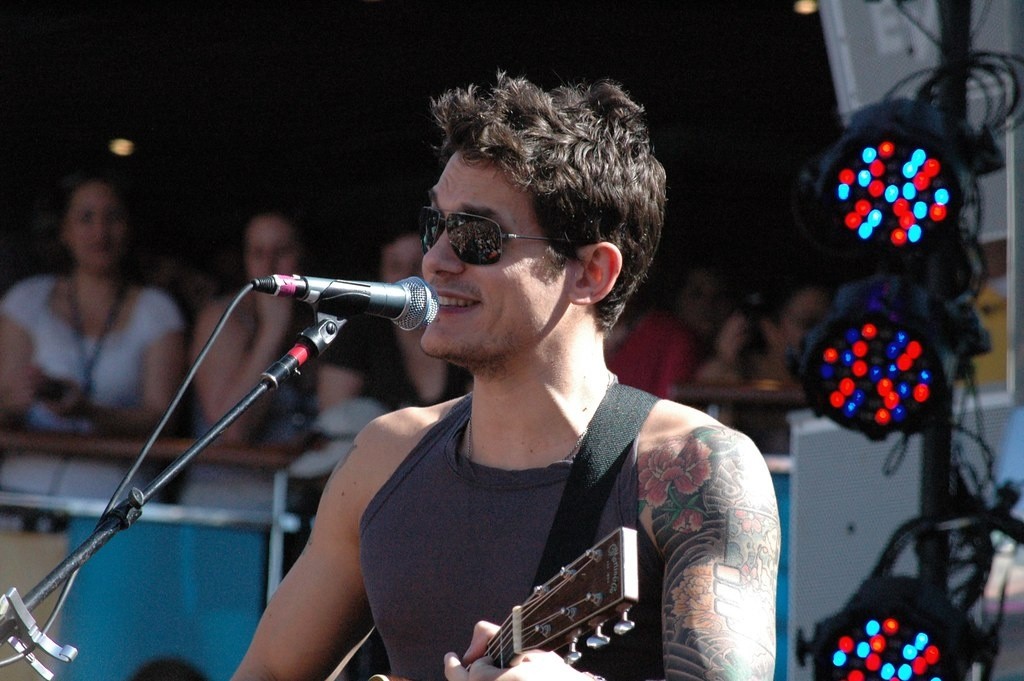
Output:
[465,370,611,465]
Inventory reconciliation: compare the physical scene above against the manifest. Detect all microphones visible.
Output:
[255,274,440,331]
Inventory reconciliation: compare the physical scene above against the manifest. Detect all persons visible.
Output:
[0,73,836,681]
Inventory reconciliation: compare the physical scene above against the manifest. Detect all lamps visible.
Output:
[798,268,976,444]
[816,96,976,276]
[795,576,993,681]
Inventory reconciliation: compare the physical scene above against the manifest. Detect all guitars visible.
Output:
[365,526,639,681]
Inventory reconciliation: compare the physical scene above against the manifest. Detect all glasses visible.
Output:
[420,206,592,265]
